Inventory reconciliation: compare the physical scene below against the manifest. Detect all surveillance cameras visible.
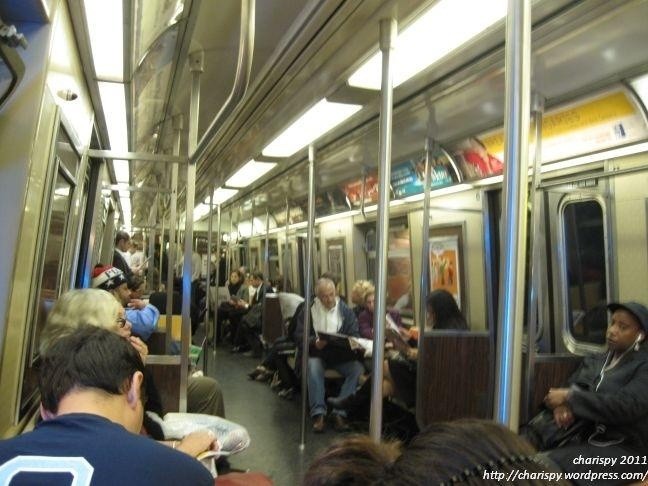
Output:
[67,90,78,101]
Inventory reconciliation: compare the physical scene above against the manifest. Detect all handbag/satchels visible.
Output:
[526,379,596,452]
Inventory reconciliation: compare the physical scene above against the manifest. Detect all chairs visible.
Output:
[141,283,588,436]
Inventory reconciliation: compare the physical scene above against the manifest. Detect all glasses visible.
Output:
[117,318,127,328]
[135,288,145,295]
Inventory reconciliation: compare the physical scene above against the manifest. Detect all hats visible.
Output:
[607,301,648,339]
[92,263,129,290]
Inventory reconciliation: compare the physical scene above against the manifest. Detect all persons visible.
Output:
[516,300,648,486]
[0,326,216,486]
[39,223,569,486]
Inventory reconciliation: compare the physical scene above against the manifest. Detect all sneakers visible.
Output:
[247,365,293,397]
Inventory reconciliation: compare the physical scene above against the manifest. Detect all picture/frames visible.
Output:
[325,236,348,302]
[421,221,471,329]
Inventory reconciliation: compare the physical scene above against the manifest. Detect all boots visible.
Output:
[327,374,371,410]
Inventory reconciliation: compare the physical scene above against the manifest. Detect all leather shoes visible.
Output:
[312,414,326,432]
[330,414,352,432]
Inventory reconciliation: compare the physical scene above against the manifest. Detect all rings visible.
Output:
[563,413,567,418]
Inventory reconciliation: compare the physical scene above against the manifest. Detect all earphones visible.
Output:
[636,333,642,341]
